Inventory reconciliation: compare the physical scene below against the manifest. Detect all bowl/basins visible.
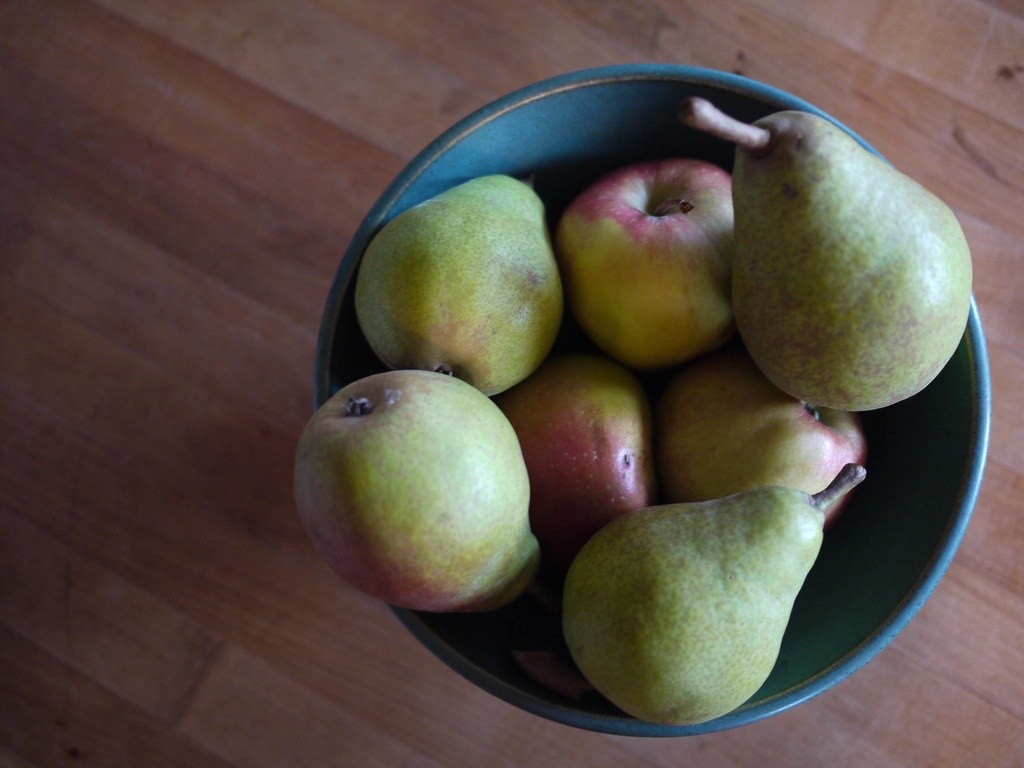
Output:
[310,63,994,737]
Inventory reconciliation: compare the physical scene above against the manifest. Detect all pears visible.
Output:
[291,95,973,724]
